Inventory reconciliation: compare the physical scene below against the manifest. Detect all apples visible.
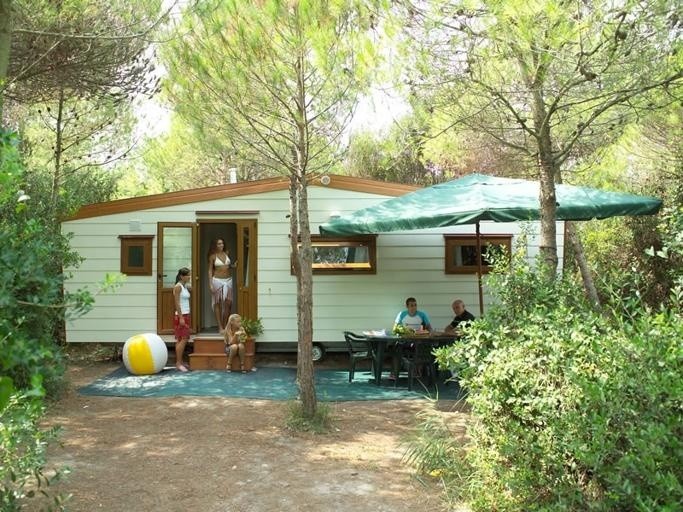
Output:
[396,324,414,335]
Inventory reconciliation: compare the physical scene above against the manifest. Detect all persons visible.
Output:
[444,299,476,335]
[389,297,433,381]
[221,313,249,374]
[172,266,191,373]
[207,237,239,333]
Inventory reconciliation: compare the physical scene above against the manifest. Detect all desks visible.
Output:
[364,333,458,387]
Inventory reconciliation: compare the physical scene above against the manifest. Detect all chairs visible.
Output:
[343,331,377,382]
[401,341,437,391]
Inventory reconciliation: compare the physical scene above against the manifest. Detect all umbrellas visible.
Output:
[319,171,664,317]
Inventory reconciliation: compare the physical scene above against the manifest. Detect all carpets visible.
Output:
[74,365,473,401]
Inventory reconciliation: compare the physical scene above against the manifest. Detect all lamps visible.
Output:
[321,176,331,186]
